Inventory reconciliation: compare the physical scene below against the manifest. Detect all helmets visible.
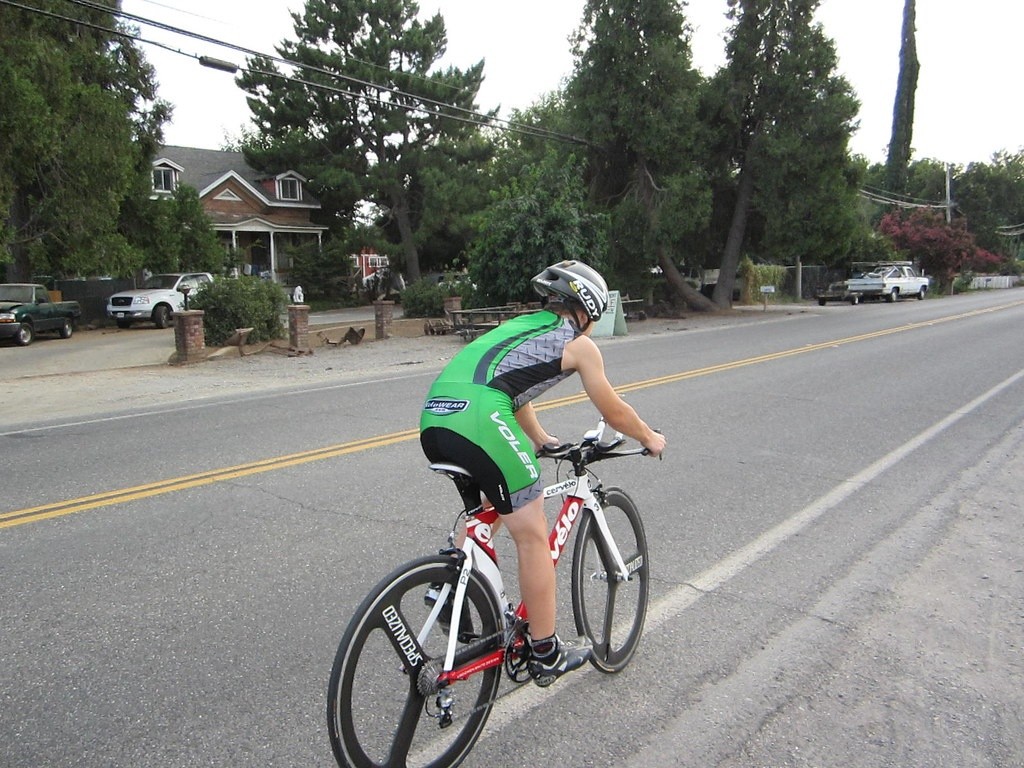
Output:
[530,257,610,323]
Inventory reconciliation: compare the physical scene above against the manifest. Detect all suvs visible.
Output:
[107,270,215,329]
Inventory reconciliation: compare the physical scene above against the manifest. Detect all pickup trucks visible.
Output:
[846,265,930,304]
[0,282,82,347]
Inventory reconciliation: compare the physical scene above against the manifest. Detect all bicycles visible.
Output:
[322,413,667,768]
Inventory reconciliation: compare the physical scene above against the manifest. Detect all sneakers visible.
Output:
[529,634,593,687]
[424,578,474,644]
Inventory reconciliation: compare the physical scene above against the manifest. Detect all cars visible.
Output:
[816,278,863,305]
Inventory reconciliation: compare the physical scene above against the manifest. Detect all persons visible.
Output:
[291,282,305,305]
[418,257,668,689]
